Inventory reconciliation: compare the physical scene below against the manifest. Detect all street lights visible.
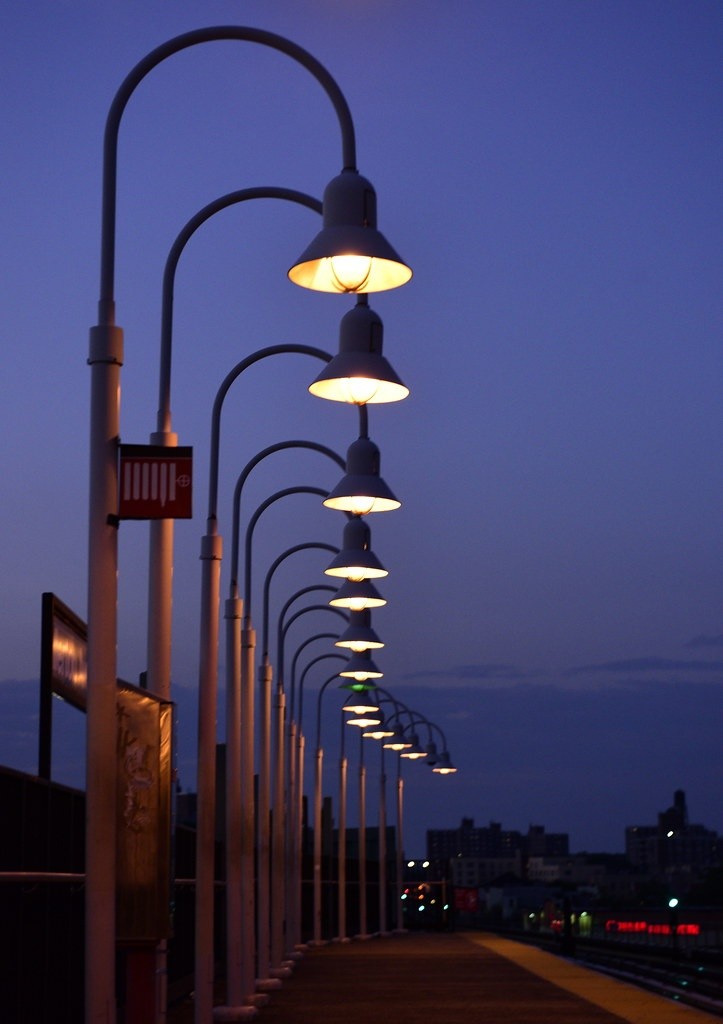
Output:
[193,343,402,1023]
[143,187,412,1024]
[81,24,418,1024]
[221,438,462,1018]
